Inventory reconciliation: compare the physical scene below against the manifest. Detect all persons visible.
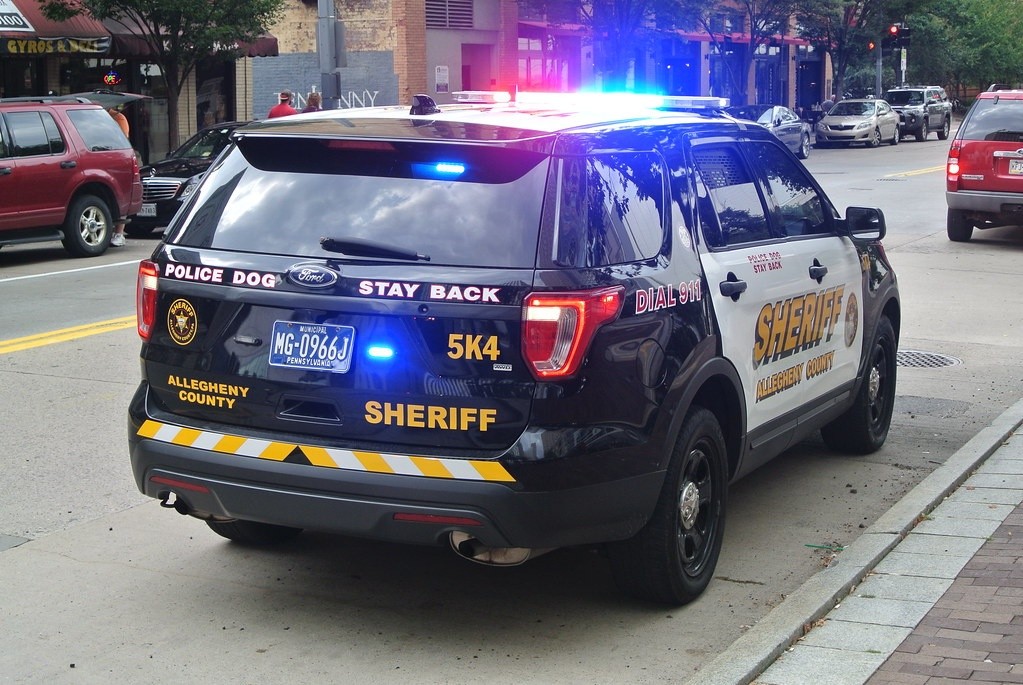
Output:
[105,108,129,246]
[302,92,321,113]
[266,91,297,119]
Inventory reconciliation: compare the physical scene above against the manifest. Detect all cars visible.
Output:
[943,83,1023,243]
[122,120,254,234]
[815,96,905,148]
[726,104,813,159]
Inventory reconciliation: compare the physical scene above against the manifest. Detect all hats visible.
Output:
[280,89,293,100]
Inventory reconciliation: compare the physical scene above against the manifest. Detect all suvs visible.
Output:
[125,80,902,609]
[885,84,953,141]
[0,88,154,256]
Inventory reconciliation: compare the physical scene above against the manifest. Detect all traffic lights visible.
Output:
[900,27,911,48]
[867,40,877,62]
[888,24,899,51]
